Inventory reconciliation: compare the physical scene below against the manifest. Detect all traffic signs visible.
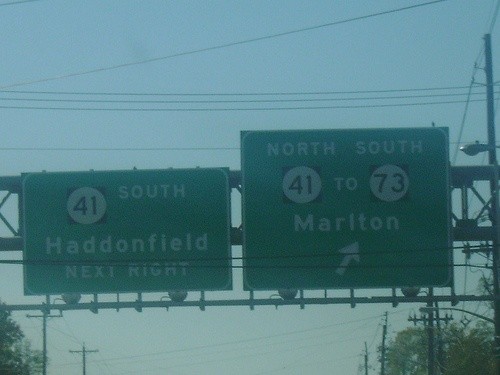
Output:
[19,166,233,297]
[241,127,454,292]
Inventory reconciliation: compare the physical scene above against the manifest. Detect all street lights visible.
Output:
[419,307,500,375]
